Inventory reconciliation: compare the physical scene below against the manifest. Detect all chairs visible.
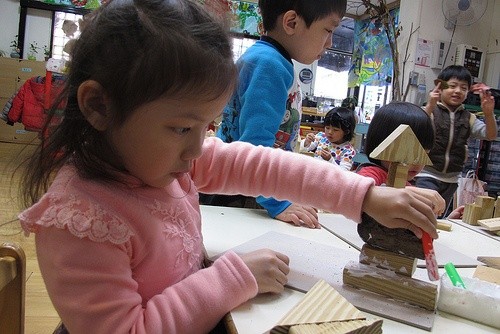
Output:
[0,240,25,333]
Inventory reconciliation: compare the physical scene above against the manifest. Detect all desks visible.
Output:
[200,206,500,334]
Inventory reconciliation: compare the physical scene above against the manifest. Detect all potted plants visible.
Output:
[42,44,52,62]
[0,50,8,57]
[29,41,39,62]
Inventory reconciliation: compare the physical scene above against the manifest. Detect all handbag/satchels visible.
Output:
[462,178,488,205]
[457,170,483,208]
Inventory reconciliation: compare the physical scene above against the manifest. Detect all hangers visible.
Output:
[43,65,57,82]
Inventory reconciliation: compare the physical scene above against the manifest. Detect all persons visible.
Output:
[354,101,465,220]
[297,97,360,172]
[18,0,439,333]
[408,66,498,222]
[199,0,348,229]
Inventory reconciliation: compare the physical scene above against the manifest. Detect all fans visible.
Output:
[442,0,489,71]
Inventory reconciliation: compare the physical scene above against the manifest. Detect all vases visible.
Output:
[11,53,20,59]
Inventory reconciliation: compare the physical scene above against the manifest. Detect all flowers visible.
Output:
[10,34,22,53]
